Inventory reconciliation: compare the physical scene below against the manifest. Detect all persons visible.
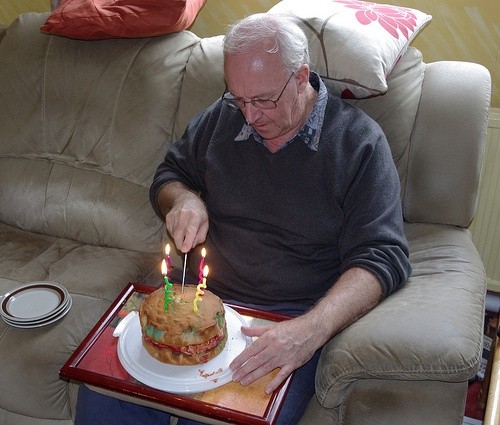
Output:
[74,11,412,425]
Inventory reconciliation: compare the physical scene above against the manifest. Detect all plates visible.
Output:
[0,281,73,328]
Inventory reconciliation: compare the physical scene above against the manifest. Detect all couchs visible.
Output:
[0,0,492,425]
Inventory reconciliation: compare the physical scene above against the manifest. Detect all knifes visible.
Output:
[181,252,187,293]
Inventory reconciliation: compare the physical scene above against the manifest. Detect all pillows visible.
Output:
[41,0,207,41]
[266,0,433,100]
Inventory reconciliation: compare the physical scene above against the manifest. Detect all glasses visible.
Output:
[222,72,294,109]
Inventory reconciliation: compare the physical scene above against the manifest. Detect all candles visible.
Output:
[158,244,210,315]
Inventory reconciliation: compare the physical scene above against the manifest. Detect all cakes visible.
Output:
[139,283,228,366]
